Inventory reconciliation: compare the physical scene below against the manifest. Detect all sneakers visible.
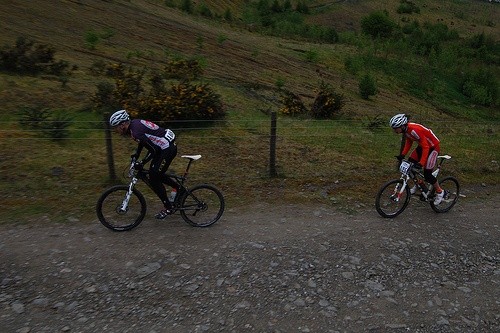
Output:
[433,189,444,205]
[154,210,168,219]
[168,196,174,202]
[409,184,421,194]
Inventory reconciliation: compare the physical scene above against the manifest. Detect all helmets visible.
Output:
[109,109,130,126]
[388,113,408,128]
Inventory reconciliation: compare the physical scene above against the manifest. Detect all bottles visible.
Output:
[168,188,177,202]
[418,179,427,190]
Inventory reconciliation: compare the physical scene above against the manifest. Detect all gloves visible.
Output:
[417,170,425,180]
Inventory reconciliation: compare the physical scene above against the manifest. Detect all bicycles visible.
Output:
[96,154,225,231]
[375,154,460,219]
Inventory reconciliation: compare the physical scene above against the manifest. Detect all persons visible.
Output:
[389,113,447,205]
[110,110,188,219]
